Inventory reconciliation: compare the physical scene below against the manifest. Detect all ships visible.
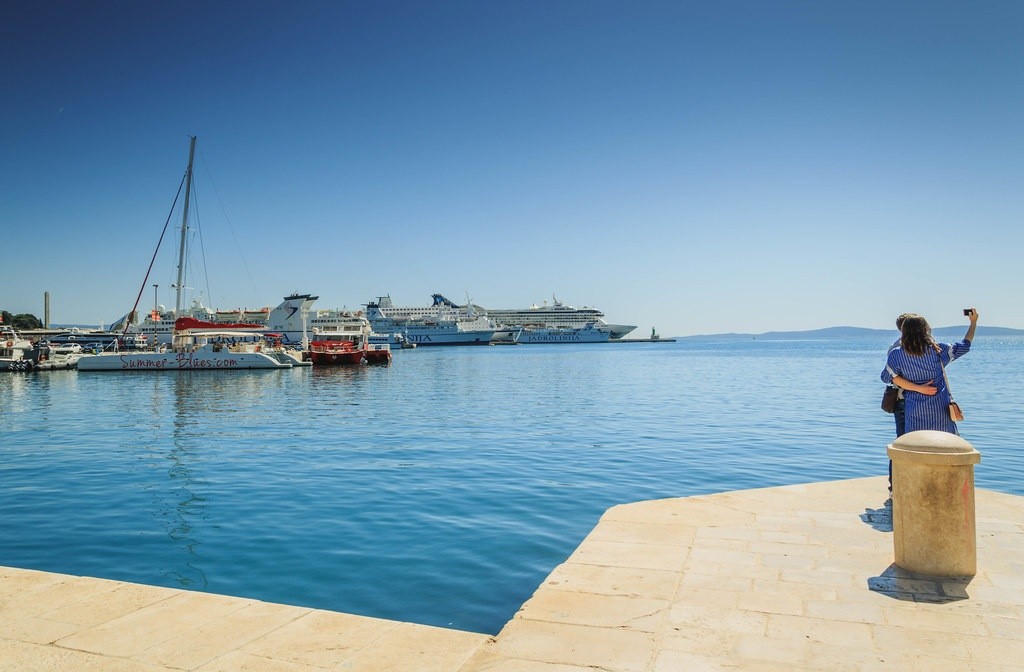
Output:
[359,293,639,339]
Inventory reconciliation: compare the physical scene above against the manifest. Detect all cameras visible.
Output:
[964,309,973,316]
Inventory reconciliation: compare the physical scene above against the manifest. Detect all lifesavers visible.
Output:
[7,341,13,348]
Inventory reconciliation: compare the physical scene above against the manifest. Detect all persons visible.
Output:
[880,306,980,499]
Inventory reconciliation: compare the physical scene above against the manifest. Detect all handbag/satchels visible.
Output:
[881,382,900,413]
[949,400,964,421]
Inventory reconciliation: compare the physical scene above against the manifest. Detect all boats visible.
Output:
[311,305,402,349]
[425,290,524,344]
[38,320,148,352]
[516,320,610,344]
[368,343,393,369]
[651,326,659,339]
[400,331,417,348]
[309,339,364,365]
[0,326,77,370]
[174,294,372,354]
[362,300,497,346]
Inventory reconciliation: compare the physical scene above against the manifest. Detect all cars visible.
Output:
[54,343,82,355]
[82,342,104,354]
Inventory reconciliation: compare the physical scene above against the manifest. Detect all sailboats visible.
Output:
[77,133,317,369]
